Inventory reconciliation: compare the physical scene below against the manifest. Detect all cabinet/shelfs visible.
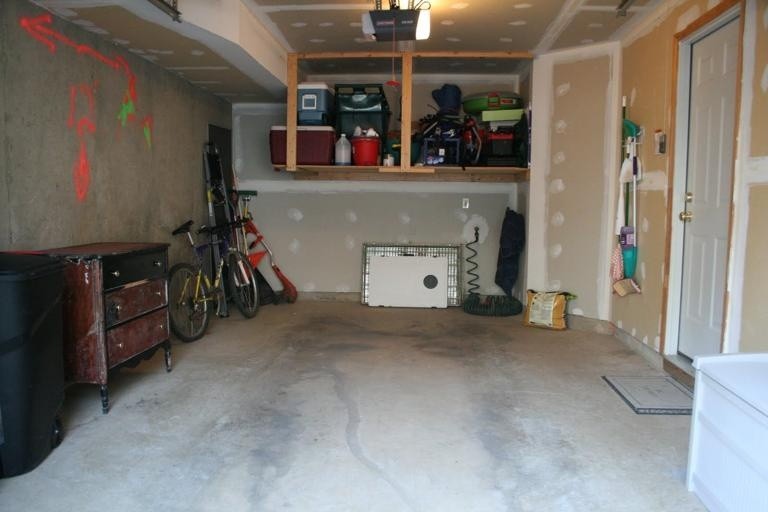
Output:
[683,354,767,512]
[34,241,172,415]
[273,47,534,184]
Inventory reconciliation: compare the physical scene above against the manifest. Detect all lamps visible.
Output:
[361,0,433,42]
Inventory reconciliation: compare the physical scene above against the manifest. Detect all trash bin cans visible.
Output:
[0,250,67,478]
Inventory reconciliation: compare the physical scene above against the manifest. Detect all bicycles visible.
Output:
[165,219,262,344]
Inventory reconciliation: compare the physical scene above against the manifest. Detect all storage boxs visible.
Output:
[268,79,337,164]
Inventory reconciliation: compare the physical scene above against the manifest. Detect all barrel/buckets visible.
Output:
[352,136,381,166]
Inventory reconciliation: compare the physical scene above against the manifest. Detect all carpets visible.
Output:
[601,374,694,415]
